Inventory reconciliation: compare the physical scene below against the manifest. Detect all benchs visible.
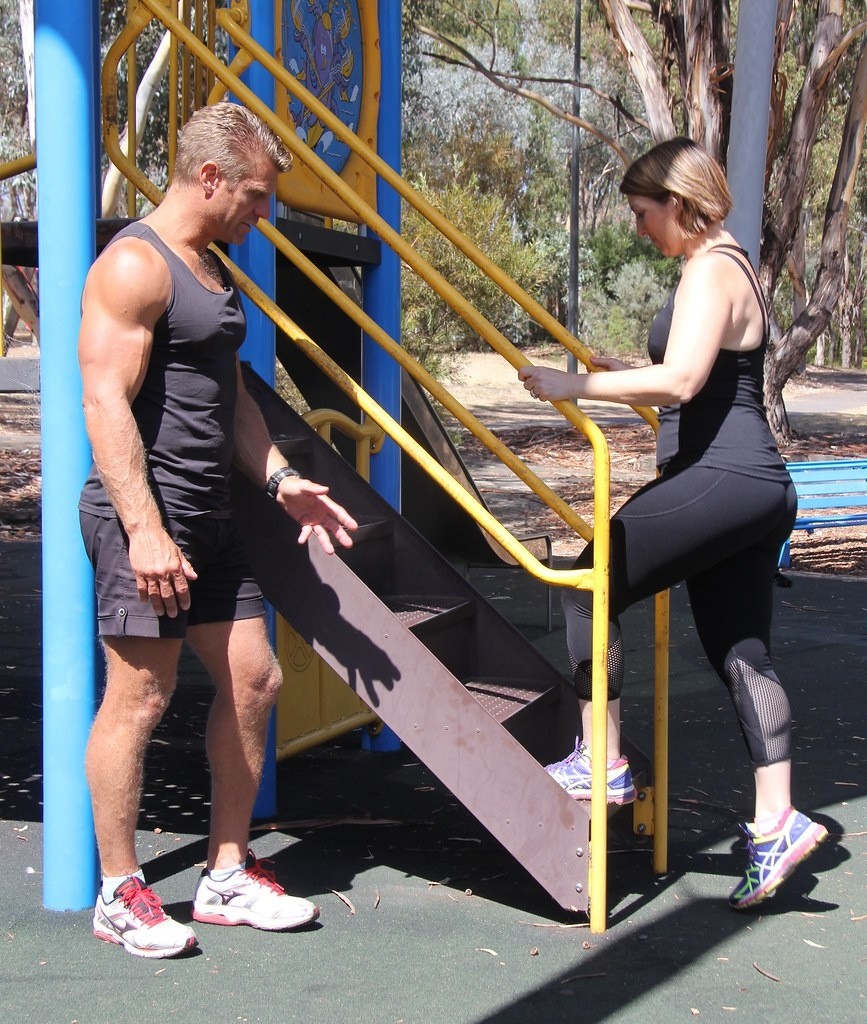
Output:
[778,459,867,567]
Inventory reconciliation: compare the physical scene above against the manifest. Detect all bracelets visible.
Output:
[264,467,302,501]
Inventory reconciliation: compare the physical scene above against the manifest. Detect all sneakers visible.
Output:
[91,878,196,957]
[542,734,638,805]
[192,848,322,931]
[728,807,829,910]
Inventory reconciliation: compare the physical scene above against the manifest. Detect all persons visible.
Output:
[80,102,358,958]
[519,137,829,907]
